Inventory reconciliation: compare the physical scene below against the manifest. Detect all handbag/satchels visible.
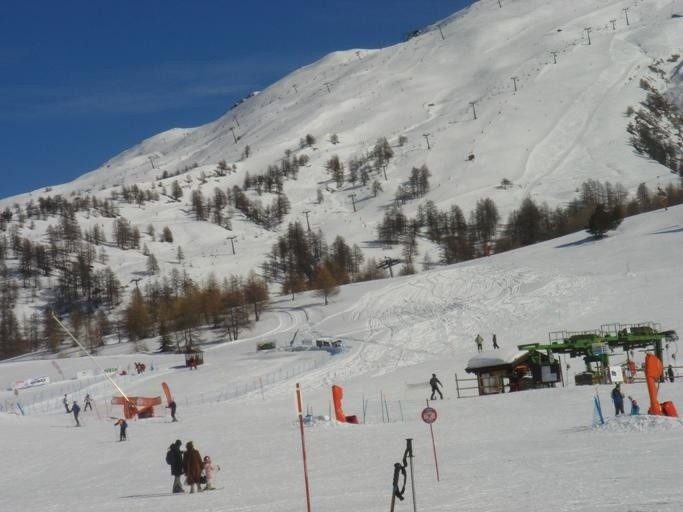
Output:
[199,475,207,484]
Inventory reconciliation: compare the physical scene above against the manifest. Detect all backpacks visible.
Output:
[166,449,174,465]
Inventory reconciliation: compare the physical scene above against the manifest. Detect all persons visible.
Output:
[630,400,639,415]
[492,334,499,349]
[474,334,484,353]
[203,455,216,490]
[189,356,196,370]
[610,383,625,414]
[83,394,93,411]
[181,441,203,493]
[429,373,443,400]
[69,400,80,426]
[165,401,177,422]
[165,439,184,493]
[113,418,128,441]
[63,393,70,413]
[668,364,674,382]
